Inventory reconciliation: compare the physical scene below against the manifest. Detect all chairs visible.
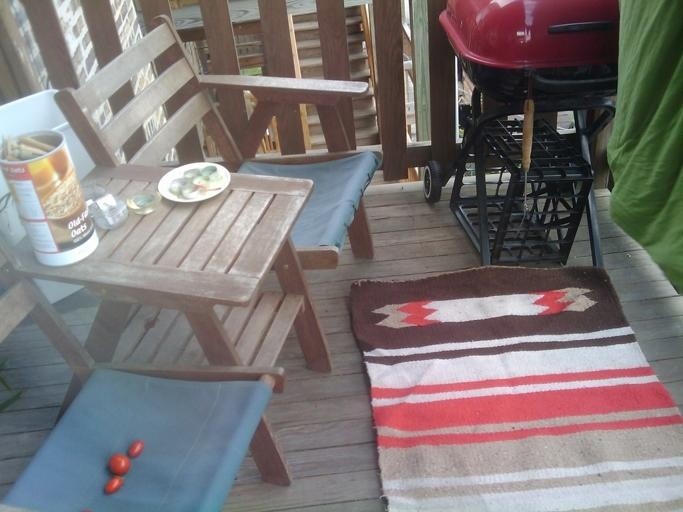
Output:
[53,13,377,375]
[2,236,294,510]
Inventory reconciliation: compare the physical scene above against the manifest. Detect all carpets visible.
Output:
[350,268,681,508]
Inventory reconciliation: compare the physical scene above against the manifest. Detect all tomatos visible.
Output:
[128,440,144,457]
[105,476,123,493]
[109,454,130,475]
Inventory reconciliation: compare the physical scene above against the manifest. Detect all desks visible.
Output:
[4,162,315,375]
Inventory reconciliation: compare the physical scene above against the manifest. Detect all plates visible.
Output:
[157,162,231,203]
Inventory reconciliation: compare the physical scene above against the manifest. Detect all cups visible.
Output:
[127,190,161,215]
